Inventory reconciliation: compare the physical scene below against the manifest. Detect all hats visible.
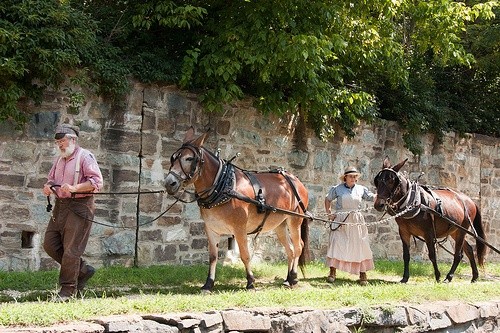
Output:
[55,124,80,139]
[339,166,361,181]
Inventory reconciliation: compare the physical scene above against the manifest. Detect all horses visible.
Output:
[165,128,310,294]
[371,155,488,284]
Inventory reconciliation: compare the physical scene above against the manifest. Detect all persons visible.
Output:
[325,166,378,285]
[43,124,103,302]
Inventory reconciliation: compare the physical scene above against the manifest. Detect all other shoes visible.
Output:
[78,265,94,290]
[54,295,74,302]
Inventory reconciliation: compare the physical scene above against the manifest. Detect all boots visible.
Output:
[328,267,336,283]
[360,272,367,286]
